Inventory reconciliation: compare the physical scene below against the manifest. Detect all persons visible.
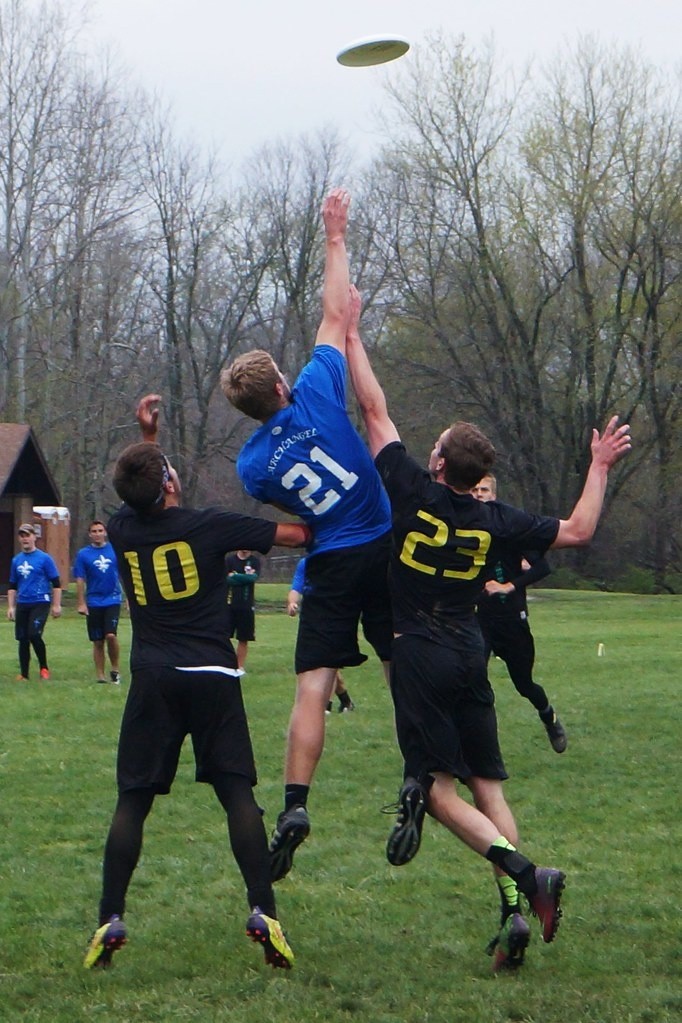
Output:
[225,550,260,673]
[8,524,62,680]
[220,189,428,884]
[73,522,129,684]
[287,558,355,716]
[83,394,310,970]
[469,474,567,754]
[346,284,631,971]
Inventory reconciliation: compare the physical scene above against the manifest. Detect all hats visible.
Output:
[17,524,36,536]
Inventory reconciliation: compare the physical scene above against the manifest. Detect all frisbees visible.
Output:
[335,38,410,67]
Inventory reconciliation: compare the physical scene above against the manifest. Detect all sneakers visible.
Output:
[267,805,311,885]
[245,905,295,969]
[97,679,108,684]
[16,675,25,682]
[339,701,354,714]
[83,915,128,969]
[110,670,120,684]
[325,700,334,714]
[542,712,567,753]
[380,776,426,867]
[485,913,529,972]
[39,668,49,680]
[527,866,566,943]
